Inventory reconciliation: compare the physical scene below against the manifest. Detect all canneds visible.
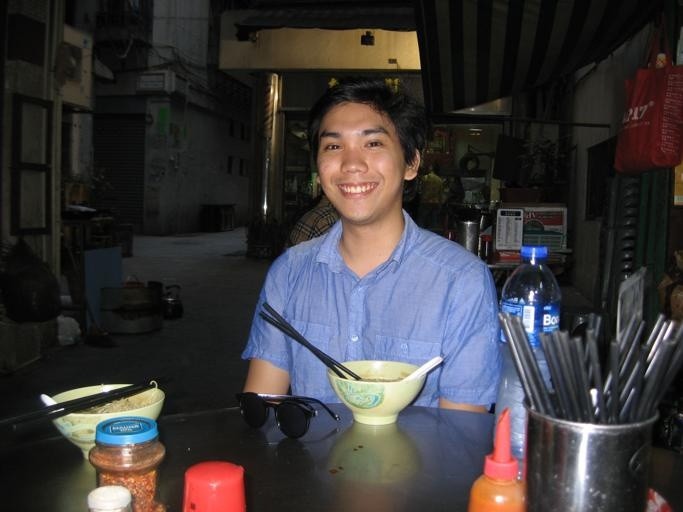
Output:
[481,235,492,258]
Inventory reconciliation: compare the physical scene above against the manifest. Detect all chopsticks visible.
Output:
[257,304,362,382]
[0,382,156,438]
[497,309,680,420]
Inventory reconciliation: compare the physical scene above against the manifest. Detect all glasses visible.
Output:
[235,392,339,439]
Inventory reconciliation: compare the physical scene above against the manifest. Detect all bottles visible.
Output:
[465,411,530,511]
[496,247,563,450]
[89,419,165,512]
[480,234,493,257]
[478,208,492,232]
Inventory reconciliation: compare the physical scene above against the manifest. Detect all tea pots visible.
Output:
[160,285,184,317]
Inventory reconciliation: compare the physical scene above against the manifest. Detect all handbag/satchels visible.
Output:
[614,64,682,172]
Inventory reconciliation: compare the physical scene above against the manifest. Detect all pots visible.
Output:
[97,286,163,334]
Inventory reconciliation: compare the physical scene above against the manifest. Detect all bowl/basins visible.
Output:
[45,384,168,460]
[326,359,426,427]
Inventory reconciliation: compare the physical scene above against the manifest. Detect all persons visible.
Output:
[282,193,341,254]
[240,79,504,415]
[416,163,443,204]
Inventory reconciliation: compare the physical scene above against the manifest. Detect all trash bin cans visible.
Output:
[67,217,122,312]
